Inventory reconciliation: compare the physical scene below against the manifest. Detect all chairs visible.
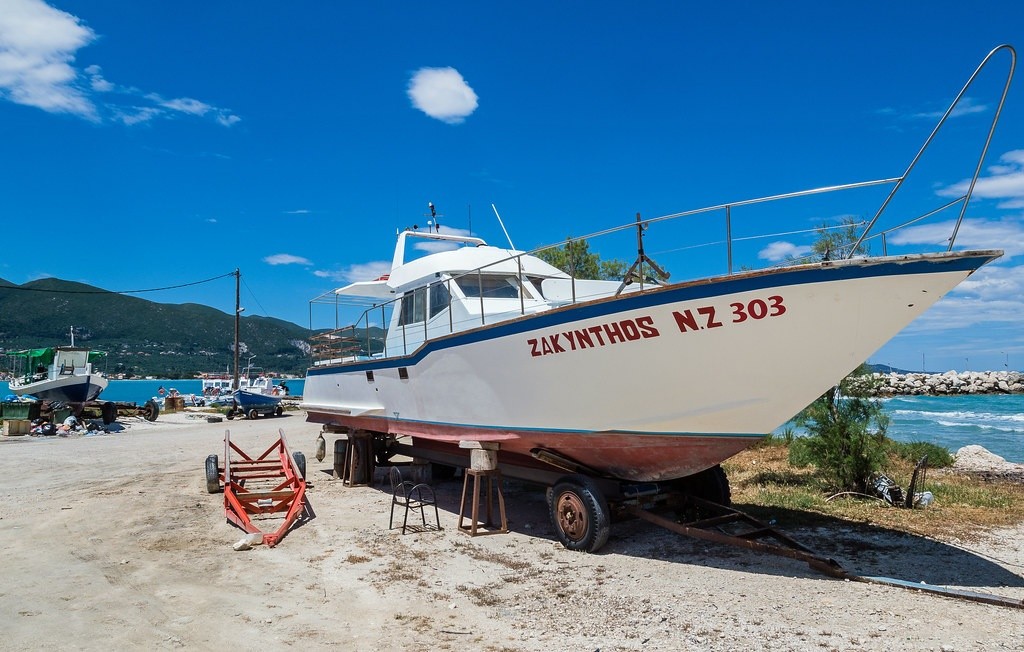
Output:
[390,466,441,535]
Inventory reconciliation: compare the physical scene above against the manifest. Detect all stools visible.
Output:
[458,469,507,537]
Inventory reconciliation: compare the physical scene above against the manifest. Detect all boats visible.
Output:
[232,377,287,412]
[9,324,109,403]
[299,42,1017,500]
[152,386,233,407]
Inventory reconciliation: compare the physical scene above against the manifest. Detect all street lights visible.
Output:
[247,355,257,378]
[234,307,245,412]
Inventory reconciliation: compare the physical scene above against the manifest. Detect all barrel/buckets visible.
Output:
[174,397,184,410]
[165,398,174,411]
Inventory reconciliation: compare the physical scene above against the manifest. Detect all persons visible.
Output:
[63,411,80,432]
